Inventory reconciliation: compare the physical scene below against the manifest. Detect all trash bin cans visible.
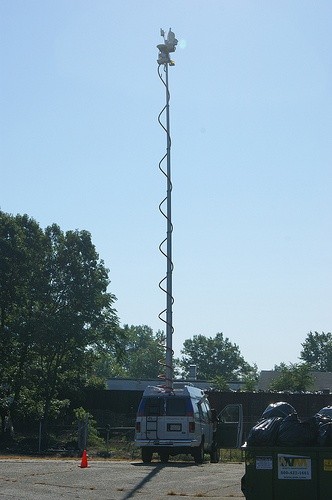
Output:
[317,446,332,500]
[240,439,277,500]
[272,444,319,499]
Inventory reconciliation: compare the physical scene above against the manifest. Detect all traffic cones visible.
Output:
[78,449,90,468]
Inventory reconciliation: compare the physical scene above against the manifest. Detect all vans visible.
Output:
[133,381,244,464]
